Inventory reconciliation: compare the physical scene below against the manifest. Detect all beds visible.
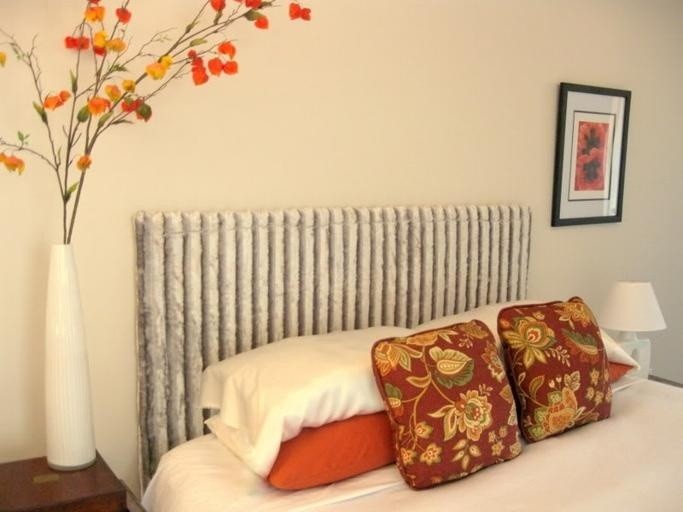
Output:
[135,204,683,512]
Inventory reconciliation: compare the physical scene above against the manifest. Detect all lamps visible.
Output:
[599,281,667,380]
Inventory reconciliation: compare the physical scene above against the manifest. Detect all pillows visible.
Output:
[372,319,522,490]
[498,296,612,442]
[267,408,396,490]
[409,300,640,370]
[198,320,422,476]
[608,360,633,385]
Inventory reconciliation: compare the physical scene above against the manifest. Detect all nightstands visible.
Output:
[0,448,128,512]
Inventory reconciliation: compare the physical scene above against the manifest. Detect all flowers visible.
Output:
[0,0,310,245]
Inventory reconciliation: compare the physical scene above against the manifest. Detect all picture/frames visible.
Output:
[552,83,631,227]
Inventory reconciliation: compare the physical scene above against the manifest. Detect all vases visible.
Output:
[45,245,96,471]
